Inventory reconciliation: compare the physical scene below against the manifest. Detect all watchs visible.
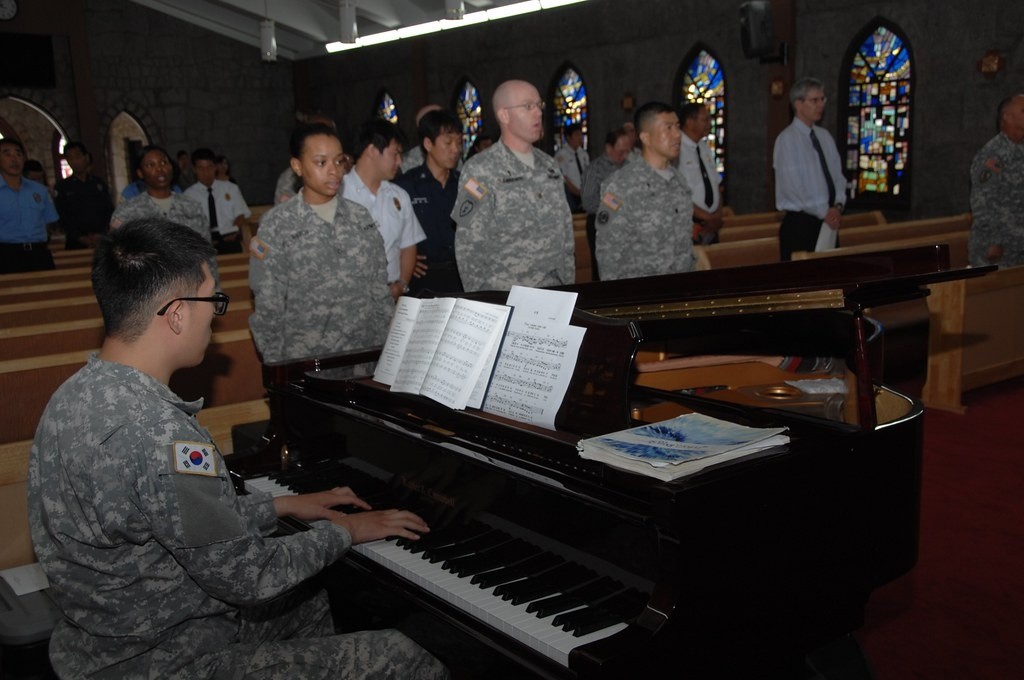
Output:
[397,279,409,294]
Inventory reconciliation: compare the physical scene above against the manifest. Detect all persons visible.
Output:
[342,118,427,302]
[580,129,630,281]
[595,101,698,281]
[451,80,575,292]
[773,76,847,260]
[396,104,463,176]
[473,134,492,152]
[0,137,59,274]
[181,147,252,255]
[555,124,590,214]
[55,141,115,250]
[110,145,211,244]
[25,219,450,680]
[968,94,1024,267]
[391,110,464,299]
[670,103,724,246]
[621,122,641,162]
[247,121,396,364]
[274,115,336,207]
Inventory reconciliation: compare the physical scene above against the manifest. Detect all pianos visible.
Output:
[213,240,955,680]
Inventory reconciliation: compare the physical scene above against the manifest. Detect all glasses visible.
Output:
[157,292,230,315]
[502,101,545,110]
[803,97,828,104]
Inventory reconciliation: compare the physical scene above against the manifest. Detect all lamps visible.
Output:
[260,0,278,60]
[339,0,361,43]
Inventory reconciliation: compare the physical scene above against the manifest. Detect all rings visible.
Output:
[831,217,836,221]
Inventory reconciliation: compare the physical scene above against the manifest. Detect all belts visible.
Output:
[212,232,238,245]
[0,242,46,251]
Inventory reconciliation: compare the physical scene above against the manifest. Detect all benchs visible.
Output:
[0,211,1024,436]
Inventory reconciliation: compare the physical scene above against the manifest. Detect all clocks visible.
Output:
[0,0,21,21]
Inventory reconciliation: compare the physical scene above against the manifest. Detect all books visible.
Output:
[576,412,790,482]
[372,294,515,412]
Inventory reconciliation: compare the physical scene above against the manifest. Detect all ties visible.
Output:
[207,186,218,240]
[696,146,714,208]
[575,152,583,174]
[809,129,836,206]
